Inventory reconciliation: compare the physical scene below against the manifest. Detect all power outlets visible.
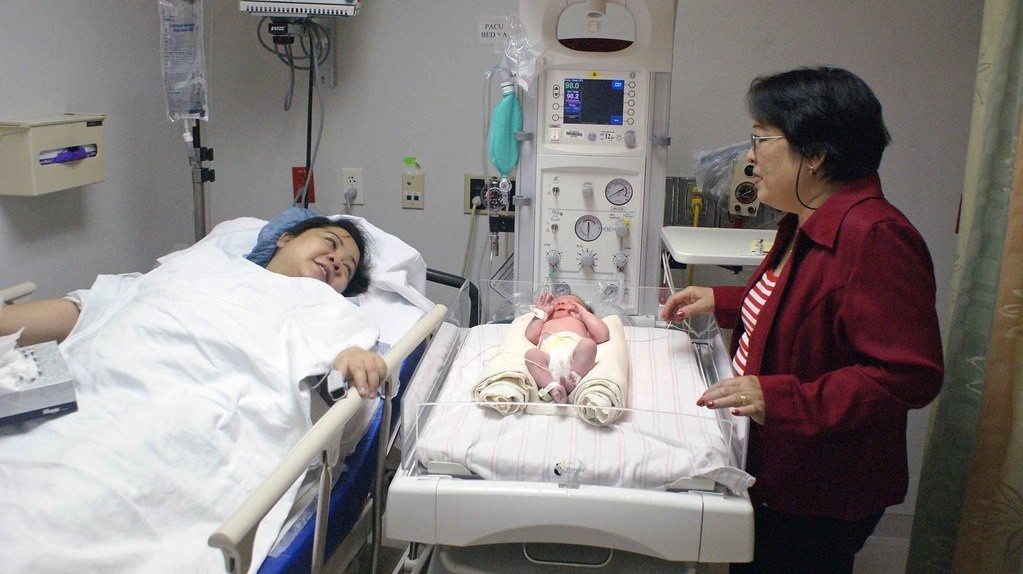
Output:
[292,166,315,203]
[342,169,364,205]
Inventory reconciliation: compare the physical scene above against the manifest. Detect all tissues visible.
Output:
[0,325,77,428]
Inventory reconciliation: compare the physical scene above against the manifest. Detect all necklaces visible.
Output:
[783,229,798,265]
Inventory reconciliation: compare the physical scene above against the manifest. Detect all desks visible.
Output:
[660,226,777,267]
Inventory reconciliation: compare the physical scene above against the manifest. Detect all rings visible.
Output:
[740,395,747,406]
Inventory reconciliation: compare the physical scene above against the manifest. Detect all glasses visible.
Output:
[749,132,788,152]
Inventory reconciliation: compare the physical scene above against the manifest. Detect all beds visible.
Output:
[385,279,755,563]
[1,213,480,573]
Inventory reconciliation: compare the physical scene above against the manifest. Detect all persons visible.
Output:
[0,217,388,399]
[525,291,610,403]
[660,66,945,574]
